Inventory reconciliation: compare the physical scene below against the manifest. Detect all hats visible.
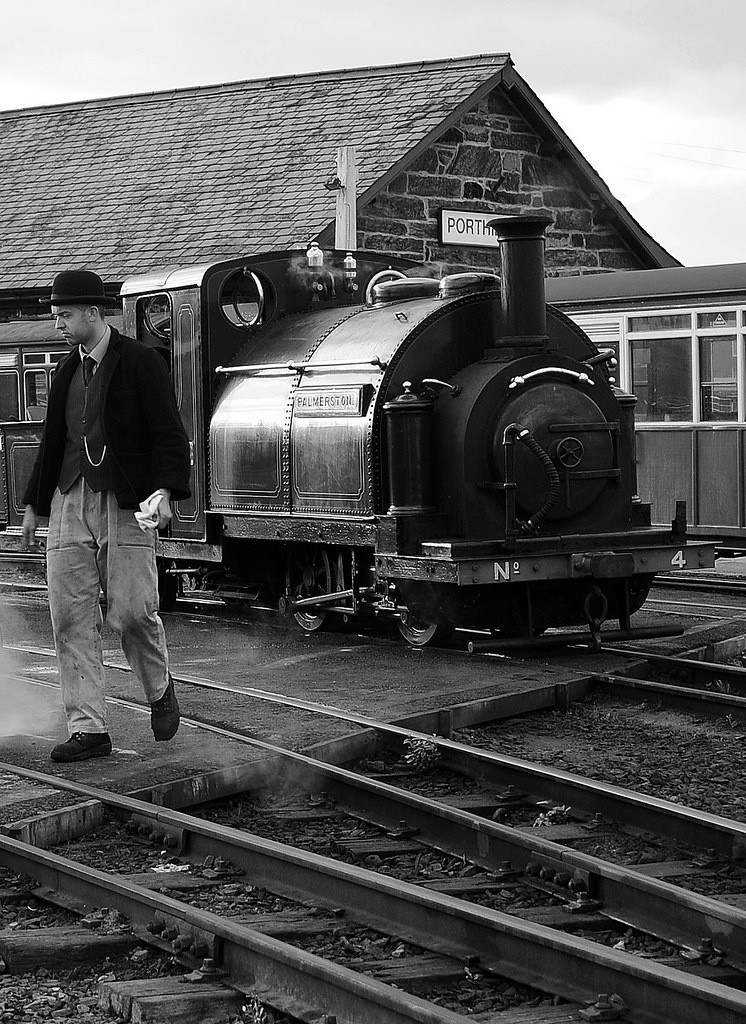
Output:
[38,271,116,306]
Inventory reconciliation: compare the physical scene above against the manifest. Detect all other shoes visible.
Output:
[150,675,180,743]
[51,731,113,763]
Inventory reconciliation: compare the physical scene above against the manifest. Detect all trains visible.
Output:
[0,214,726,655]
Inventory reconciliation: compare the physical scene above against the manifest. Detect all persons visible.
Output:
[19,270,193,763]
